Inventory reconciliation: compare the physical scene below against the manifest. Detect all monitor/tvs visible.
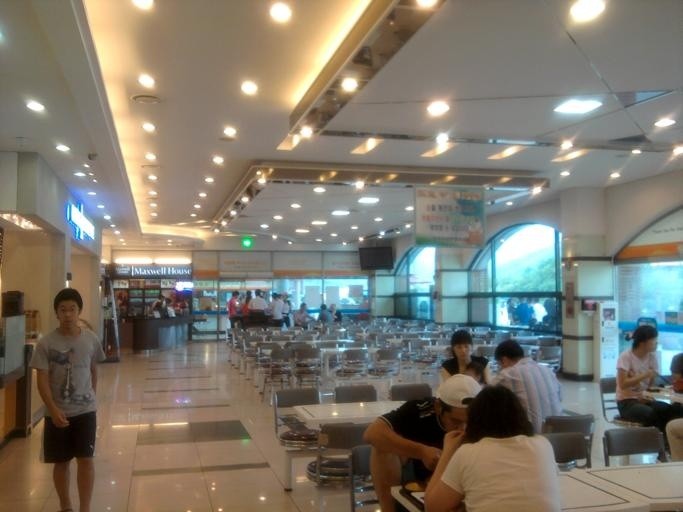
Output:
[359,247,393,271]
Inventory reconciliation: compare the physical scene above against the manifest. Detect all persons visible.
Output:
[489,338,564,435]
[505,297,556,326]
[615,324,682,465]
[361,373,485,511]
[25,287,106,511]
[226,288,343,336]
[422,384,561,511]
[149,295,170,311]
[436,329,487,388]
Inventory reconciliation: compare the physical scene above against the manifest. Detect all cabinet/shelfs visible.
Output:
[105,275,191,317]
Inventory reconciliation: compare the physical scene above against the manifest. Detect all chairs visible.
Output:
[223,308,683,512]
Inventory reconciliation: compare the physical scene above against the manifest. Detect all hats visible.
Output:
[435,373,483,409]
